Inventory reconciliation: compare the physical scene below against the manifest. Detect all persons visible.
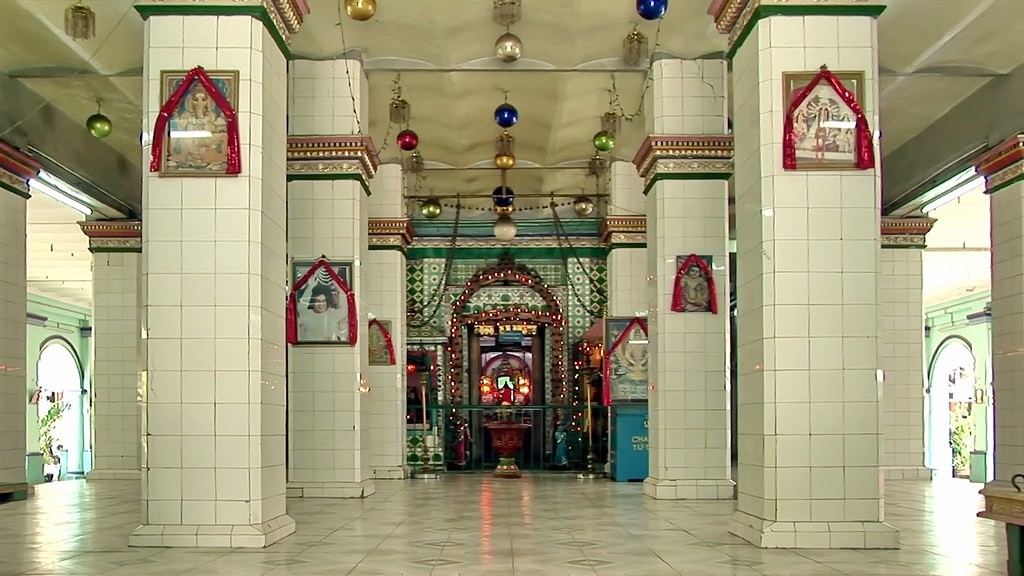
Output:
[408,391,423,424]
[299,293,342,342]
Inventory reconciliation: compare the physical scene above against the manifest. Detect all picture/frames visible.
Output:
[156,67,243,181]
[780,67,872,174]
[599,314,648,406]
[286,258,356,349]
[672,254,714,315]
[367,320,393,367]
